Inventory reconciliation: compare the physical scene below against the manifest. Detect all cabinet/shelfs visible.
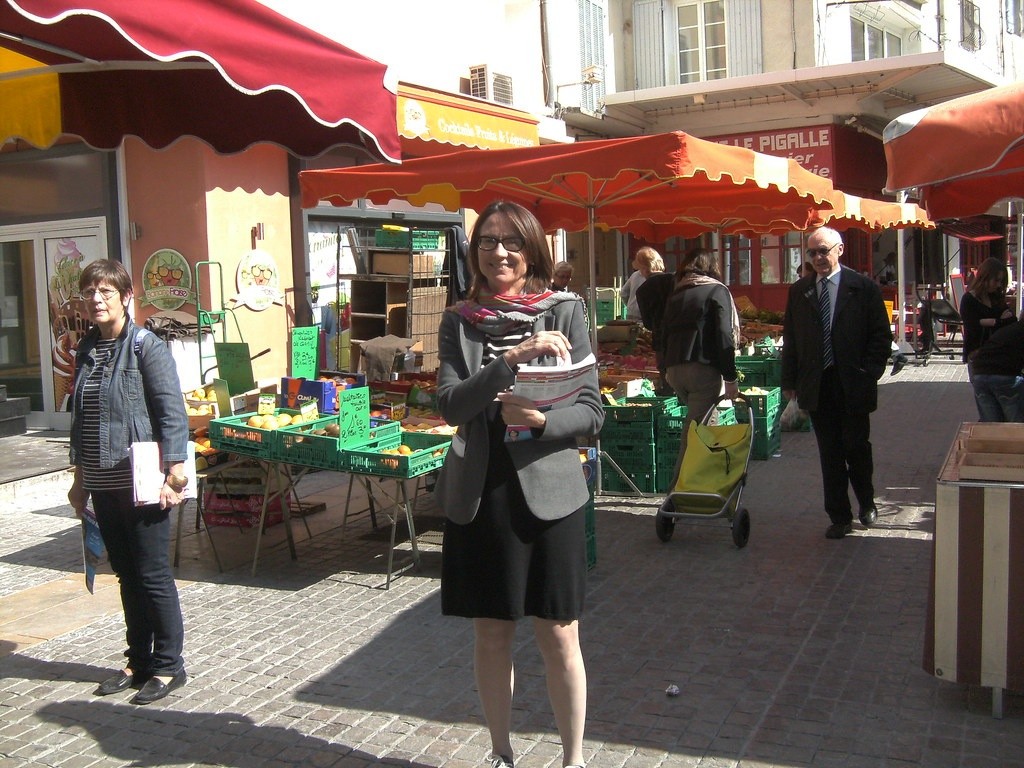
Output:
[336,224,451,373]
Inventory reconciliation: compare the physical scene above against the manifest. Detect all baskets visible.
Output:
[373,226,440,248]
[210,406,452,479]
[587,298,627,326]
[598,354,812,494]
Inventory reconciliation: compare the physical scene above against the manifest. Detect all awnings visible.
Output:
[393,84,539,157]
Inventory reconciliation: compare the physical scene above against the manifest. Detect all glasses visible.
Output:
[478,235,523,252]
[80,288,121,300]
[807,242,840,258]
[556,274,572,281]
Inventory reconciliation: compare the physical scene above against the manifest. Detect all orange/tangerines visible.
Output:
[319,376,383,418]
[192,426,220,454]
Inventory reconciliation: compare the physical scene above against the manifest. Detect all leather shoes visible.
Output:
[131,667,187,705]
[858,504,878,527]
[98,667,150,695]
[824,522,853,539]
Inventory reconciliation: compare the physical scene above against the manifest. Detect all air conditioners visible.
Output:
[469,64,513,105]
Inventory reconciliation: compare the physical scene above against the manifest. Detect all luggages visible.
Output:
[653,394,753,548]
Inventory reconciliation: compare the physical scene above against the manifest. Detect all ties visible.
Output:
[819,278,834,370]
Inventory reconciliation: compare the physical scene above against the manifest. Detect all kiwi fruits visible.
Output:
[309,424,339,438]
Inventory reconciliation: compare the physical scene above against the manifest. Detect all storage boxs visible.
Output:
[373,228,440,248]
[373,251,434,275]
[182,288,811,571]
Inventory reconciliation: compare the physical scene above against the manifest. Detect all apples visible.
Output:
[247,413,306,433]
[185,388,217,417]
[380,446,413,468]
[598,332,656,370]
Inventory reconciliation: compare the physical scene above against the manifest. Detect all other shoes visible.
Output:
[562,762,586,768]
[890,355,908,376]
[486,754,515,768]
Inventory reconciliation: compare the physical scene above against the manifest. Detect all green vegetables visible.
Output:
[741,306,781,324]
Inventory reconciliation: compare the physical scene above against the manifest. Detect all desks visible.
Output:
[250,459,443,592]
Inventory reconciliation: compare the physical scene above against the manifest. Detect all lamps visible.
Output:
[556,64,603,111]
[847,118,884,143]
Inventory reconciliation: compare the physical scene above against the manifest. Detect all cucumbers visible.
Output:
[242,264,272,280]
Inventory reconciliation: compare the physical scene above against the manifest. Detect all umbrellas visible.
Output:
[299,82,1024,368]
[0,1,401,165]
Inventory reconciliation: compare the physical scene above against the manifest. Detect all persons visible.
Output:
[959,257,1024,424]
[554,246,741,497]
[509,430,520,442]
[437,202,605,768]
[796,253,899,289]
[782,228,895,539]
[69,259,188,703]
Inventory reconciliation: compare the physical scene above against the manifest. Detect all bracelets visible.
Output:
[725,377,738,383]
[167,473,188,490]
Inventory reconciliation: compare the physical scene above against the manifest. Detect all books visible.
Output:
[504,353,596,443]
[127,440,199,507]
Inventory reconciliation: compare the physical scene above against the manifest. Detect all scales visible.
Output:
[597,320,639,343]
